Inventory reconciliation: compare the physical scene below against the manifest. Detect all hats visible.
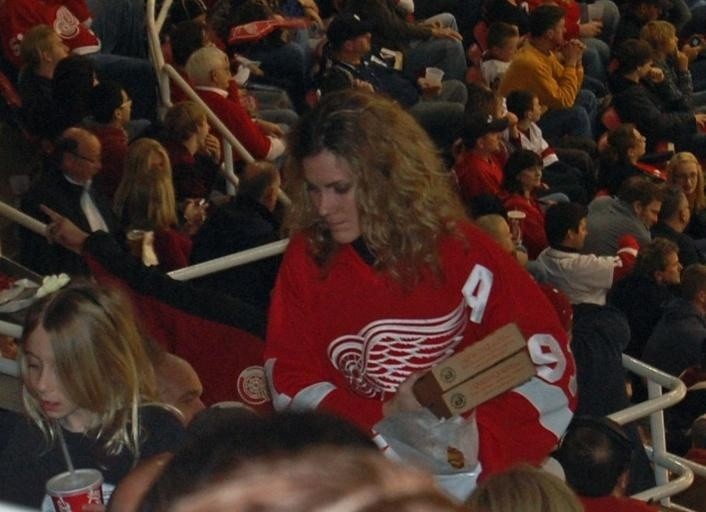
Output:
[169,0,208,23]
[323,10,380,50]
[461,108,509,132]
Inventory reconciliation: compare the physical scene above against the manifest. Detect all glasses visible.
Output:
[119,100,132,109]
[71,152,101,164]
[637,137,646,143]
[675,173,697,179]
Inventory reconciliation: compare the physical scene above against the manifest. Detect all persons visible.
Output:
[461,465,583,512]
[506,89,587,203]
[572,297,658,495]
[529,0,611,81]
[169,20,206,100]
[455,110,508,200]
[140,349,210,430]
[609,239,685,326]
[631,264,706,457]
[467,189,508,220]
[185,47,286,172]
[652,183,706,265]
[599,126,665,198]
[611,38,706,160]
[616,0,706,92]
[0,282,183,512]
[158,102,221,200]
[47,54,99,125]
[193,163,285,308]
[318,12,467,136]
[121,170,194,275]
[579,180,664,256]
[534,200,621,306]
[207,0,326,86]
[16,25,69,108]
[560,412,662,512]
[114,139,209,235]
[346,0,466,81]
[498,148,548,259]
[82,82,133,193]
[503,5,597,161]
[21,126,111,272]
[464,90,518,135]
[479,23,518,89]
[475,212,513,255]
[666,153,706,237]
[639,21,705,113]
[40,204,269,342]
[265,89,578,501]
